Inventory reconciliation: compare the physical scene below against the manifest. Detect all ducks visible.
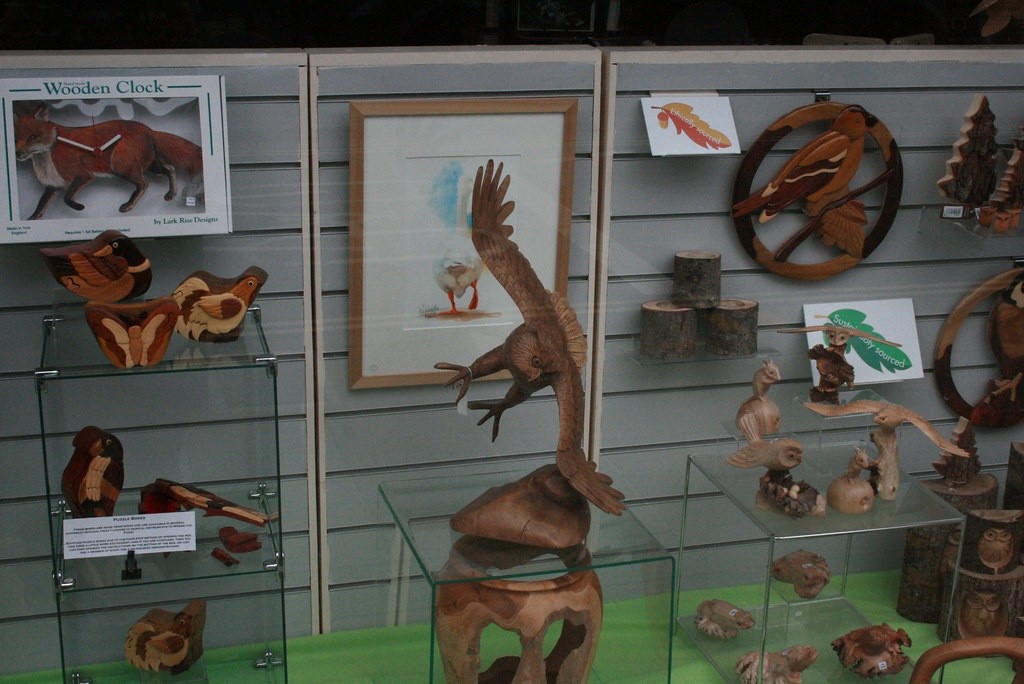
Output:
[430,173,484,314]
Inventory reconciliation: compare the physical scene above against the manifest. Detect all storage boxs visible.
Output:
[1,73,234,245]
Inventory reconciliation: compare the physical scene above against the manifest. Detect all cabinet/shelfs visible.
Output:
[36,289,290,683]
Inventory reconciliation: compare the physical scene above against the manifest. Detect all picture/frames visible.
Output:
[349,95,578,394]
